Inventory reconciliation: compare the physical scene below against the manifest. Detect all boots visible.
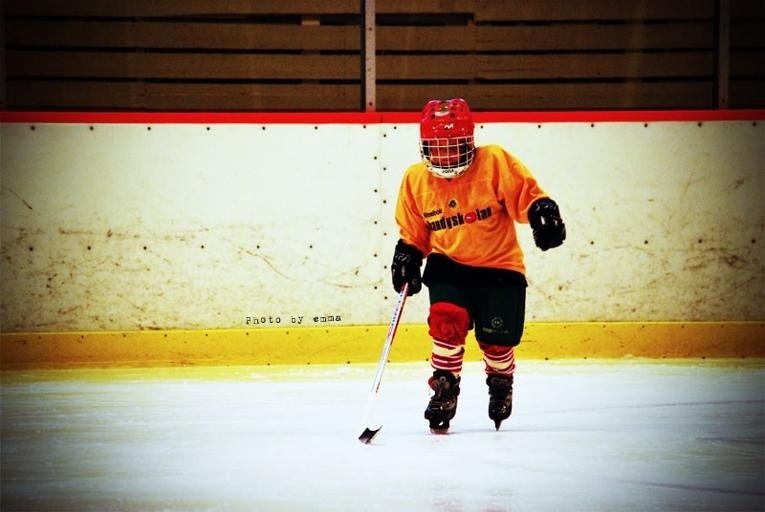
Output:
[424,367,465,419]
[484,372,515,420]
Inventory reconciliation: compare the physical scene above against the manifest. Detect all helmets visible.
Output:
[418,97,475,161]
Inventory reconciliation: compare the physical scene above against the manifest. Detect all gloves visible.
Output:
[391,239,424,296]
[527,198,567,251]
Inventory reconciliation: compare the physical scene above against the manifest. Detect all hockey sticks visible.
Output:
[359,284,408,444]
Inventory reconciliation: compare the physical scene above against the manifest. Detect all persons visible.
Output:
[388,97,565,428]
